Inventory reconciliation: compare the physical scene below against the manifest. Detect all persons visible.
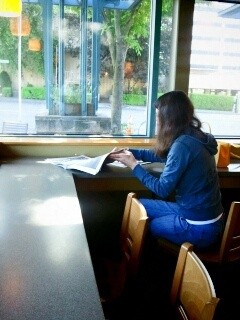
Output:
[98,90,225,297]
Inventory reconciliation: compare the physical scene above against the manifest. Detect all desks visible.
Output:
[0,158,240,320]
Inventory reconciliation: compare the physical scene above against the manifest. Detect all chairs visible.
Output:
[100,192,149,302]
[158,200,240,265]
[170,242,220,320]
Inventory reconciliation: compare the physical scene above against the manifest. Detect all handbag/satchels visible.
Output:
[214,141,229,168]
